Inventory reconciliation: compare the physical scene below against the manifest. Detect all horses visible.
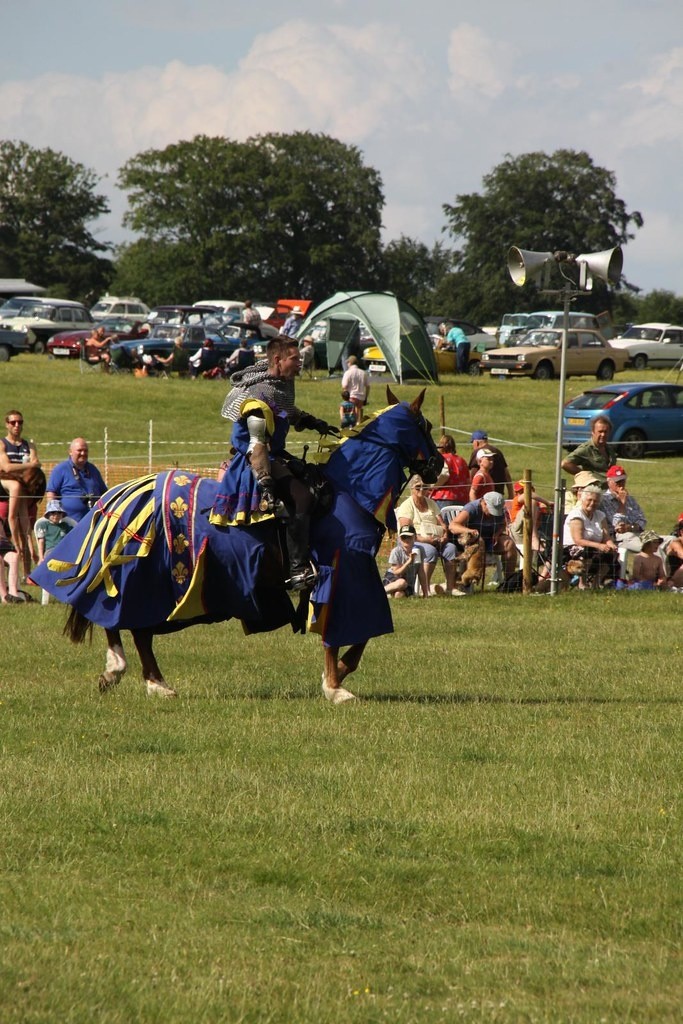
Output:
[61,383,446,705]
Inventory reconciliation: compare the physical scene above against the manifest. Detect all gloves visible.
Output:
[313,418,329,435]
[260,483,277,515]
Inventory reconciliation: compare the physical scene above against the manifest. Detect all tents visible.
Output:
[296,291,438,385]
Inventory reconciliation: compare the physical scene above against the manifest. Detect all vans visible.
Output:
[499,311,599,345]
[151,300,275,325]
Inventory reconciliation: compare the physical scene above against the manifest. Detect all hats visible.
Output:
[514,479,534,491]
[476,448,497,458]
[483,492,505,516]
[44,500,68,519]
[571,471,601,488]
[399,525,416,537]
[639,530,664,550]
[677,513,683,522]
[345,355,358,364]
[469,431,487,443]
[290,306,305,315]
[607,465,628,482]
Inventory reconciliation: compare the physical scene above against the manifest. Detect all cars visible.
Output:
[89,295,150,320]
[0,295,94,362]
[479,328,630,380]
[591,322,683,368]
[561,379,683,454]
[311,320,497,373]
[48,314,151,360]
[109,314,280,372]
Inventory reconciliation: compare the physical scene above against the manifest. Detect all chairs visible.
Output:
[394,500,628,592]
[649,392,664,406]
[568,334,578,346]
[35,516,78,606]
[80,337,314,384]
[535,334,542,341]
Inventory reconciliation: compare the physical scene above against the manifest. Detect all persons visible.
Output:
[283,306,303,338]
[209,336,340,592]
[339,355,371,431]
[243,300,261,337]
[0,409,108,604]
[84,327,119,370]
[383,416,683,599]
[439,322,471,374]
[153,336,315,378]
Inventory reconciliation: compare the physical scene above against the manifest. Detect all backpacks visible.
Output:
[496,566,539,596]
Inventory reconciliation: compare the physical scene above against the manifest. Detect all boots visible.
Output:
[283,515,335,590]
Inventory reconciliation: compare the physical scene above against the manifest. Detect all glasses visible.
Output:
[6,419,25,425]
[415,484,428,491]
[518,490,523,493]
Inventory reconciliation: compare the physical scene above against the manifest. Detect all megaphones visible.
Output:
[507,246,556,287]
[576,246,623,285]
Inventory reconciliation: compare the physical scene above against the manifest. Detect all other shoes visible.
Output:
[446,588,467,597]
[613,581,625,590]
[4,591,32,603]
